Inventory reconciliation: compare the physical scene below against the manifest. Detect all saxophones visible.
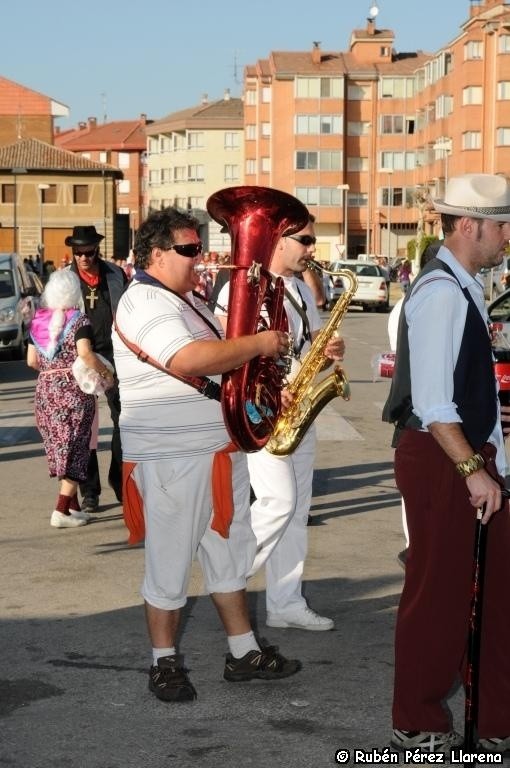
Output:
[265,262,358,458]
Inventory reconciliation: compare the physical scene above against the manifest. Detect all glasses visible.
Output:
[283,234,317,245]
[162,241,203,256]
[72,247,96,257]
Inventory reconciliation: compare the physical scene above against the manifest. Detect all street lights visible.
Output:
[433,136,452,199]
[336,183,353,257]
[376,166,394,259]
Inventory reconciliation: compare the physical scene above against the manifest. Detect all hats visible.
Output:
[65,226,104,246]
[434,173,510,223]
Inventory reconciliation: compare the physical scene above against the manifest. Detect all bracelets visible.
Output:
[455,453,485,479]
[99,369,109,380]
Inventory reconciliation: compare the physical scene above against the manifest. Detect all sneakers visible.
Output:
[474,737,508,758]
[224,646,301,682]
[266,606,335,631]
[391,727,464,757]
[148,655,197,702]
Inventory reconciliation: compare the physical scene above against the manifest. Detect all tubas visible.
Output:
[195,186,310,453]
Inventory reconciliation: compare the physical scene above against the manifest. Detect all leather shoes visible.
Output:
[81,496,99,512]
[49,511,87,528]
[108,480,123,502]
[68,509,91,521]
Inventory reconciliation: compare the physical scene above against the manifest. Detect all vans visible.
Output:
[0,251,37,357]
[354,254,394,273]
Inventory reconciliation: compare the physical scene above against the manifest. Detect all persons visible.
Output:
[387,239,507,568]
[64,225,129,511]
[27,268,115,529]
[316,255,415,297]
[213,215,346,631]
[111,205,302,702]
[381,173,510,762]
[26,251,236,306]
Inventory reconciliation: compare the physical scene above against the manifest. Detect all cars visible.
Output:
[486,285,510,354]
[26,269,44,308]
[321,259,391,314]
[479,256,510,300]
